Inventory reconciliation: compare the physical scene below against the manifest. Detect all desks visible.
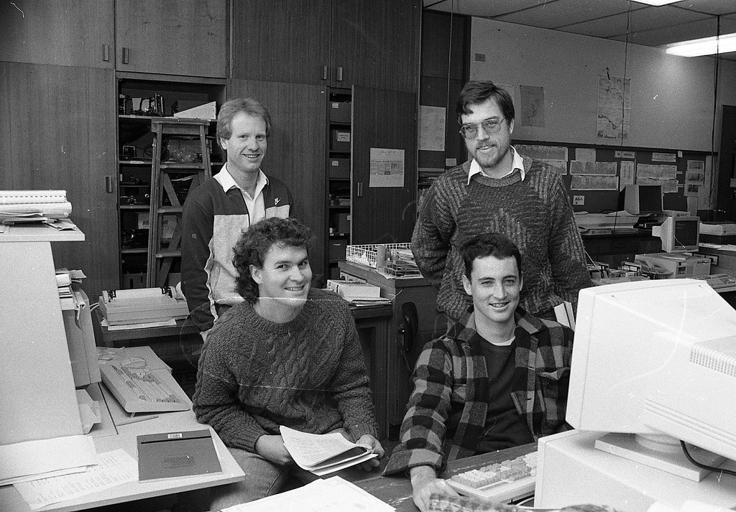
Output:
[0,347,246,512]
[339,262,453,428]
[94,300,392,447]
[226,443,536,512]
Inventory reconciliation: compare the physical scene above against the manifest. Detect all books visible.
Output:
[1,189,72,225]
[279,426,379,480]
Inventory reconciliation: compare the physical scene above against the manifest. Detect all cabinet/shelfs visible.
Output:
[228,0,423,291]
[0,0,227,346]
[418,8,471,191]
[581,235,661,269]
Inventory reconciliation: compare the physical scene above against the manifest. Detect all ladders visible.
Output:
[147,118,213,287]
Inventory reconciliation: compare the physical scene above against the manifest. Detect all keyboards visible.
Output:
[705,278,726,287]
[100,364,191,418]
[445,450,538,504]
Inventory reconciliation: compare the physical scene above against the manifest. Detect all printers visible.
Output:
[97,285,191,326]
[696,210,736,245]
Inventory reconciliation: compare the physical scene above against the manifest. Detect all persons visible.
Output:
[409,80,598,339]
[194,216,385,511]
[381,231,575,512]
[180,95,295,400]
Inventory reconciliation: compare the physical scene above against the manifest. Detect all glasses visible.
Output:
[459,117,506,139]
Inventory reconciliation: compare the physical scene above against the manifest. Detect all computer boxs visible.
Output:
[635,252,711,279]
[534,429,736,512]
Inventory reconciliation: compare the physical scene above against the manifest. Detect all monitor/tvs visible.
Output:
[565,278,736,483]
[652,216,700,261]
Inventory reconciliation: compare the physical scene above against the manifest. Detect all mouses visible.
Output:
[728,279,735,283]
[120,357,146,369]
